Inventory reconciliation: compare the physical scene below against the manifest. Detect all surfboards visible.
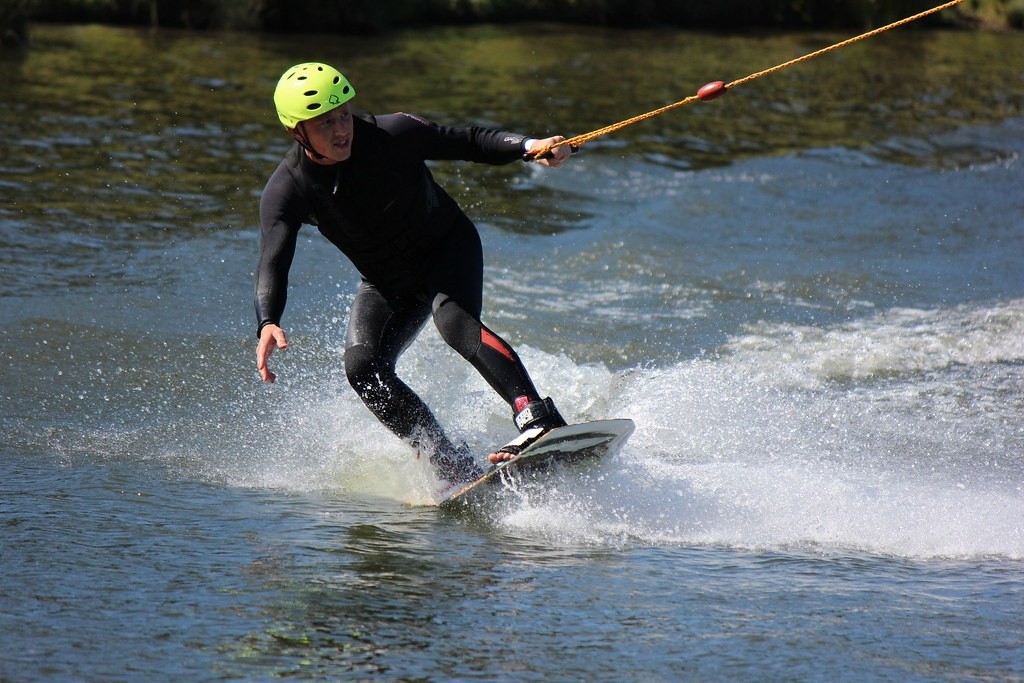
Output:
[438,418,636,514]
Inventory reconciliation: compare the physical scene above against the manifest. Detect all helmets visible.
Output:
[274,62,356,129]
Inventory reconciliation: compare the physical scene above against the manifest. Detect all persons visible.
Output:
[255,63,570,491]
